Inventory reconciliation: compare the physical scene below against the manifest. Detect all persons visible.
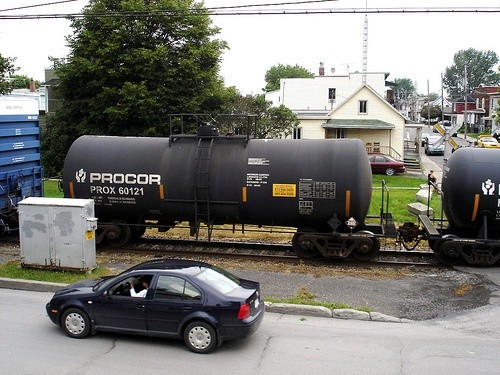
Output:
[129,276,151,298]
[428,170,437,192]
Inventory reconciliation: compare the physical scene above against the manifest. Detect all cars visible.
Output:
[368,152,406,176]
[415,133,430,147]
[473,134,500,149]
[422,135,445,156]
[432,119,457,137]
[45,257,265,355]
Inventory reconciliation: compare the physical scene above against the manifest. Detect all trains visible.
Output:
[0,91,500,270]
